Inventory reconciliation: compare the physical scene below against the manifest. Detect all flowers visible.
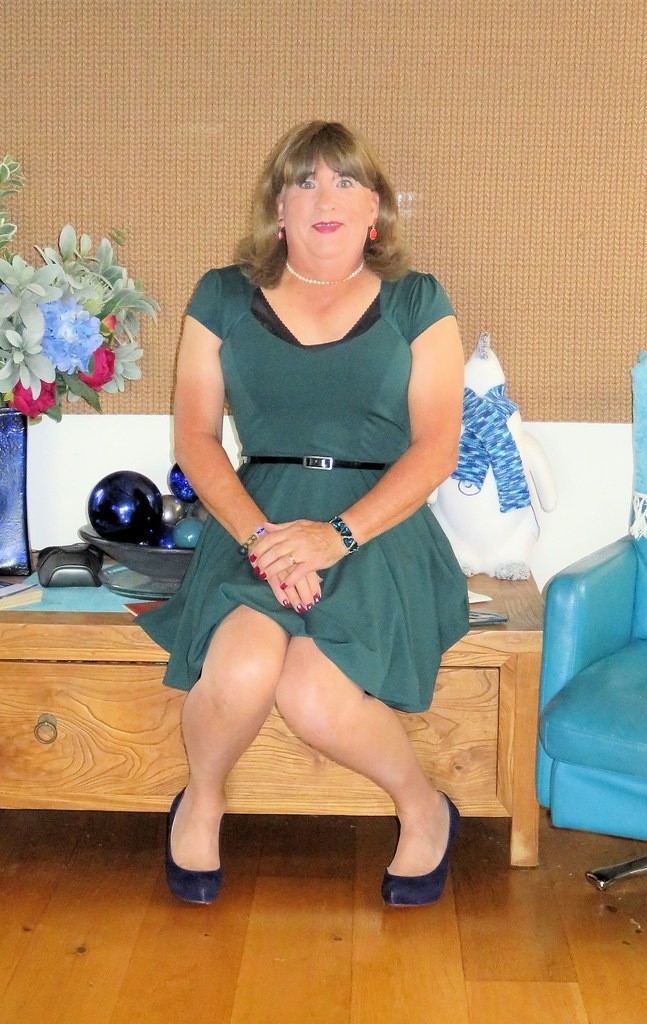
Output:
[0,153,161,422]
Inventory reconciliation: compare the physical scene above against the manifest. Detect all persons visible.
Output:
[130,122,487,908]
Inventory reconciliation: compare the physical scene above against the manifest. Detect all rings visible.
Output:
[287,553,296,565]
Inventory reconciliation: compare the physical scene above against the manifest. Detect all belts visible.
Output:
[242,455,390,470]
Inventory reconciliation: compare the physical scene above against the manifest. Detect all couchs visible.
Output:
[535,349,647,890]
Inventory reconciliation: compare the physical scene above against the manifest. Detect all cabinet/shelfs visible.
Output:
[0,549,548,868]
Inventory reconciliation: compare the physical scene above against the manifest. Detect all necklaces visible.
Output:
[285,258,365,286]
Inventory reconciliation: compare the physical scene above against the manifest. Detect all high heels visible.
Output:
[380,790,461,908]
[165,786,223,903]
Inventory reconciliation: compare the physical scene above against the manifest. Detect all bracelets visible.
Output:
[237,527,265,556]
[328,515,359,555]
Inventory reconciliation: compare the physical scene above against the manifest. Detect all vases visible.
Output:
[0,408,33,577]
